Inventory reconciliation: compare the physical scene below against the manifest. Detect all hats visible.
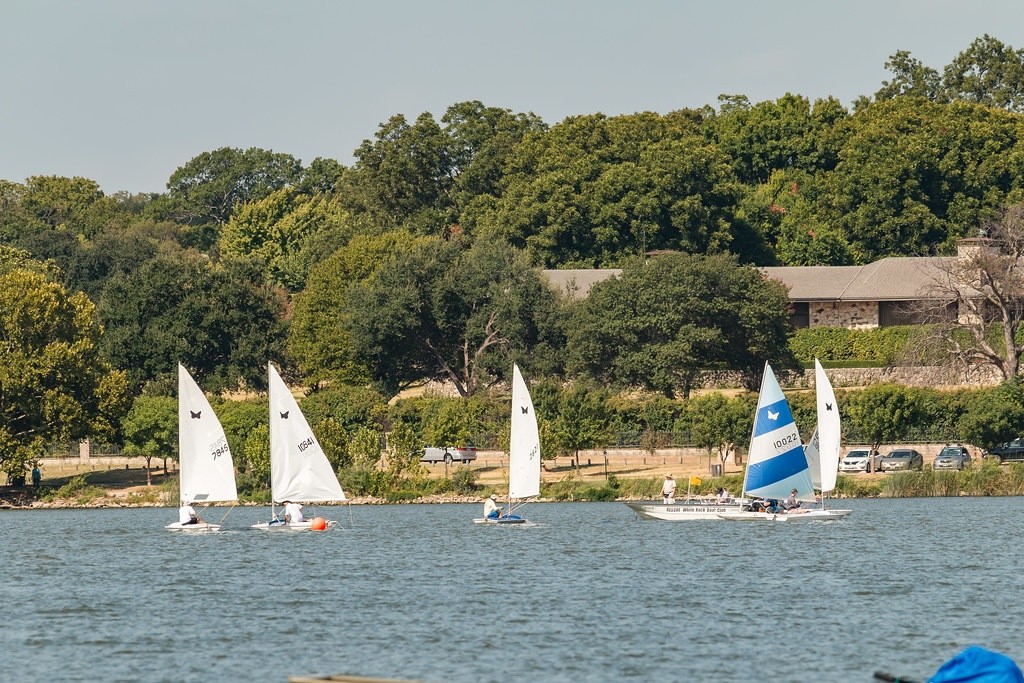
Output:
[666,472,673,480]
[282,500,292,506]
[491,494,497,500]
[183,501,190,505]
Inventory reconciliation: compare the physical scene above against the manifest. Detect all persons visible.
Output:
[787,488,802,508]
[717,487,727,503]
[32,465,41,485]
[484,494,504,518]
[660,473,676,504]
[764,498,782,513]
[180,500,197,525]
[282,501,304,522]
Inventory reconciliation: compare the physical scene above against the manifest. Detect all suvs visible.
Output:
[982,437,1024,465]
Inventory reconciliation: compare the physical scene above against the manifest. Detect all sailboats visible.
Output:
[251,363,347,531]
[475,363,540,527]
[165,361,237,535]
[718,359,817,522]
[784,357,844,521]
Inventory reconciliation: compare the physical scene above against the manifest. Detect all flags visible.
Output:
[690,476,702,486]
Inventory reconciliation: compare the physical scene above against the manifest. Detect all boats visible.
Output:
[623,499,852,520]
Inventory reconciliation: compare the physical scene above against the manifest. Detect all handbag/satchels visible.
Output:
[663,494,669,498]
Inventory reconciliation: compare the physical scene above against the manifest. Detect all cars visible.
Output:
[934,444,971,471]
[838,449,884,472]
[407,441,476,464]
[881,449,923,471]
